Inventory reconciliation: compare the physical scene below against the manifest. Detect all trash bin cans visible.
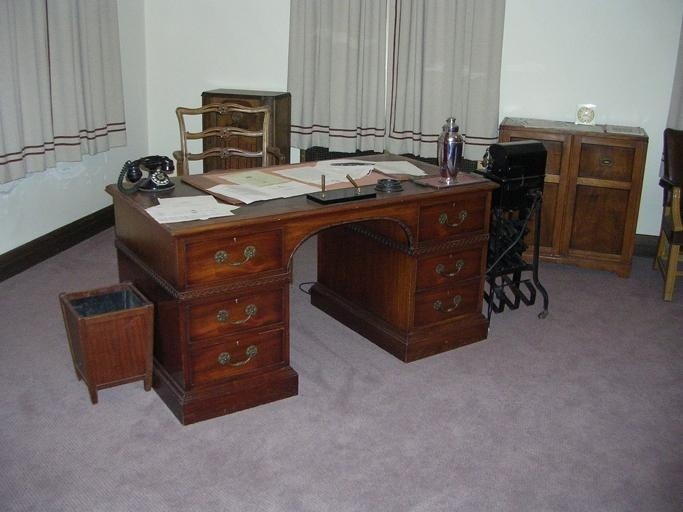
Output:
[58,280,154,405]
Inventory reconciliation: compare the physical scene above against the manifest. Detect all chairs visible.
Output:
[653,128,680,302]
[175,103,272,174]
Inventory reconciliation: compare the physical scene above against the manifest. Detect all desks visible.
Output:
[105,154,502,426]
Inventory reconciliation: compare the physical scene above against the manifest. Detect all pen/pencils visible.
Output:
[331,162,376,166]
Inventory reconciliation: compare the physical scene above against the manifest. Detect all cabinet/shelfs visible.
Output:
[202,88,293,174]
[497,117,649,278]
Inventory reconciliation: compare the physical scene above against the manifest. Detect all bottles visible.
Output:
[436,117,463,185]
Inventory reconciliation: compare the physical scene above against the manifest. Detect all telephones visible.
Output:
[126,156,175,192]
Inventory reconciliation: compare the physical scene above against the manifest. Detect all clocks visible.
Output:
[574,104,598,127]
